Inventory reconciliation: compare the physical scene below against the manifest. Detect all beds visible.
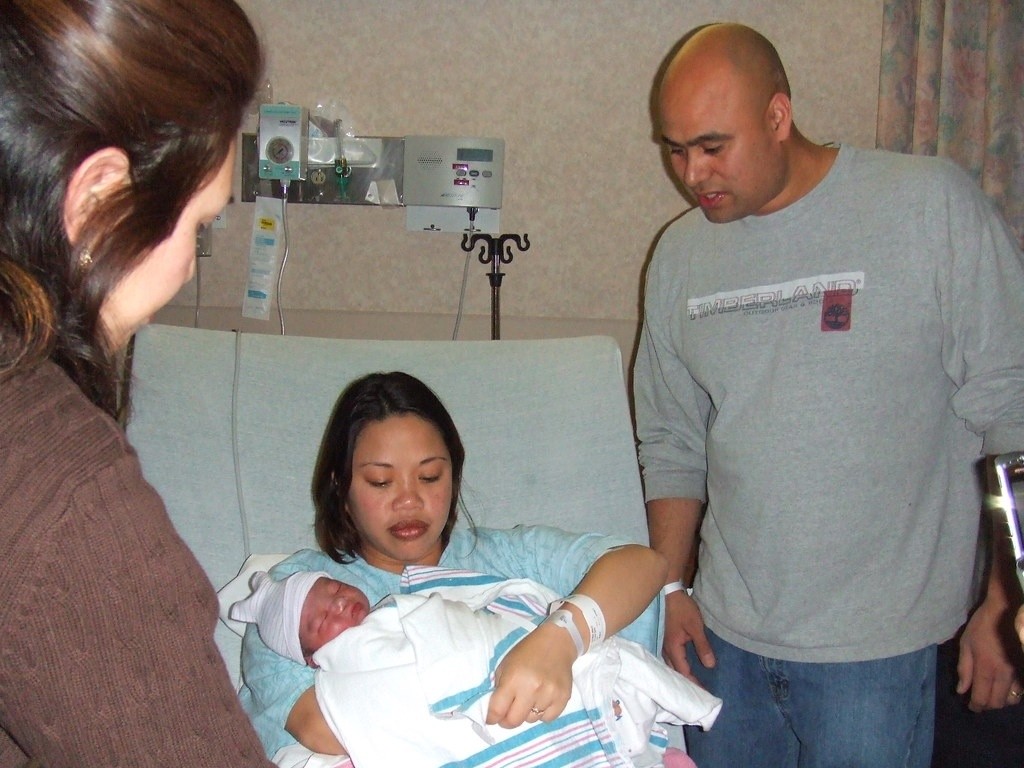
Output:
[125,320,690,768]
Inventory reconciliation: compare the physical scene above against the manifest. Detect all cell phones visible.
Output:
[994,451,1024,593]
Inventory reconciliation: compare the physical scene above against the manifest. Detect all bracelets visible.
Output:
[662,578,687,594]
[540,594,606,658]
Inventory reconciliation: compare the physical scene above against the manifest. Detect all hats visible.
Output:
[230,569,334,668]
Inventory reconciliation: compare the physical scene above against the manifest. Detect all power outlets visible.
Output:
[241,132,404,204]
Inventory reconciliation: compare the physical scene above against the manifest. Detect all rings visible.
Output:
[531,707,543,716]
[1008,688,1023,699]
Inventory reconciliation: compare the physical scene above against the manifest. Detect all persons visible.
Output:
[631,22,1024,768]
[0,0,271,768]
[240,371,698,767]
[229,570,723,767]
